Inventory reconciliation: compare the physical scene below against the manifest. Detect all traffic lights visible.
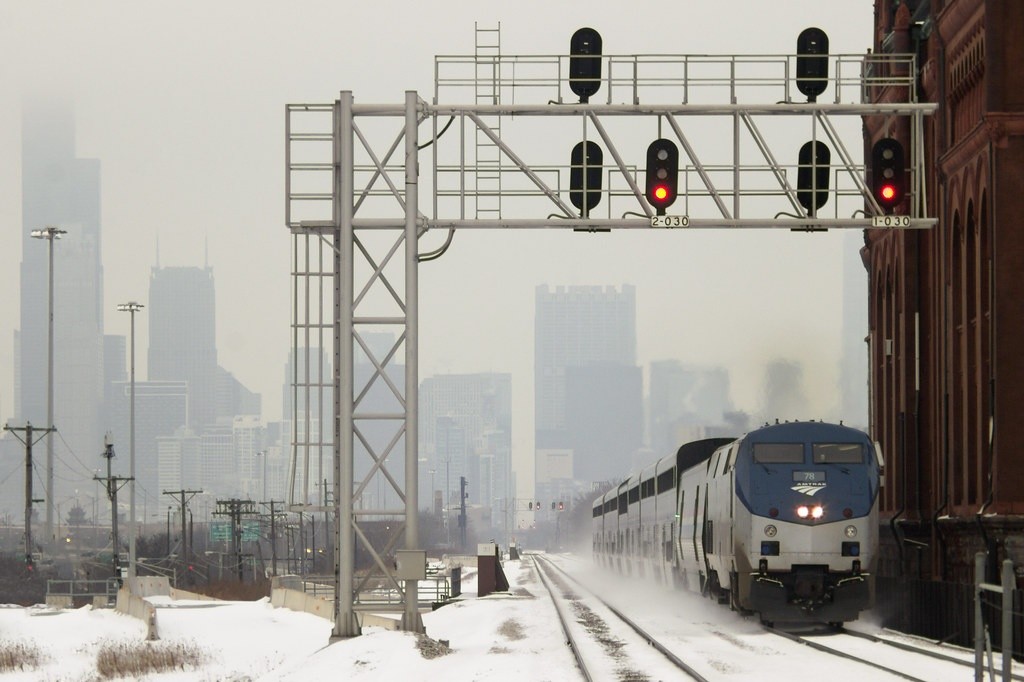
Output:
[536,502,541,510]
[559,502,563,511]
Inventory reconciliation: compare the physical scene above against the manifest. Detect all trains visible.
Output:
[588,418,887,633]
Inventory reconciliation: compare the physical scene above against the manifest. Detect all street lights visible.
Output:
[31,226,69,556]
[872,139,906,204]
[117,300,145,587]
[645,138,679,210]
